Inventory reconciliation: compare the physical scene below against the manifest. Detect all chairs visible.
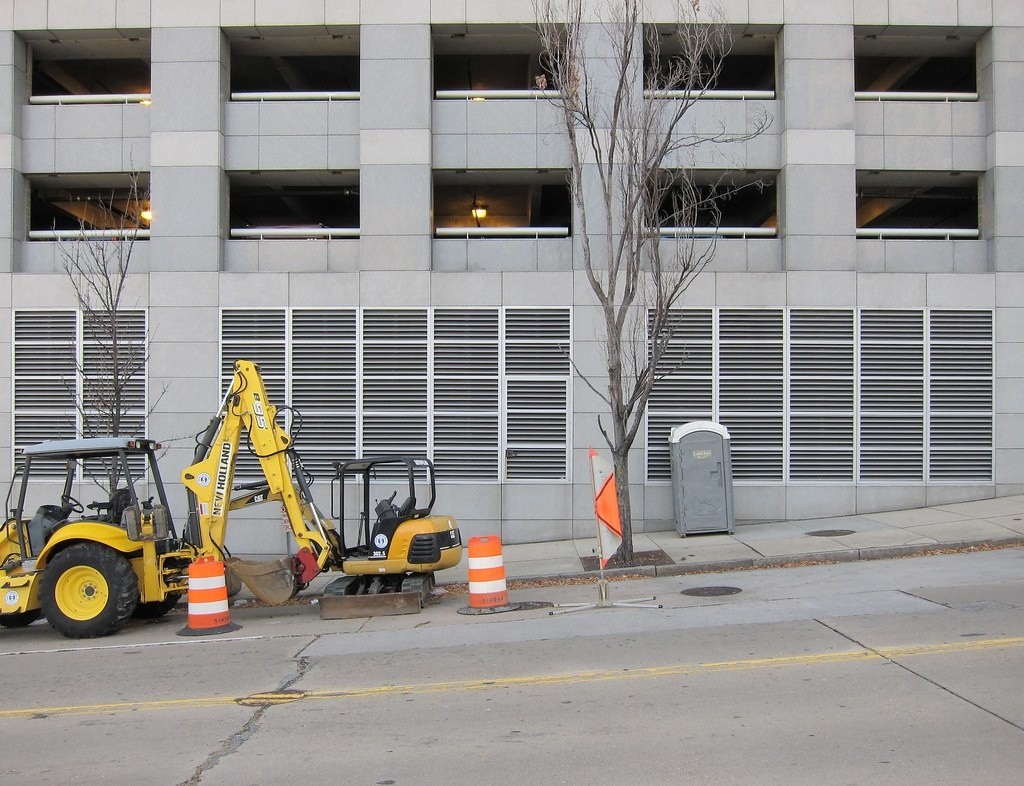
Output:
[87,489,131,523]
[396,497,417,515]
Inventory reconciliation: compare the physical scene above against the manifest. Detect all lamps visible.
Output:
[471,205,488,217]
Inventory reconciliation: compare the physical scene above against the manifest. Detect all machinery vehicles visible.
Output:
[0,359,464,642]
